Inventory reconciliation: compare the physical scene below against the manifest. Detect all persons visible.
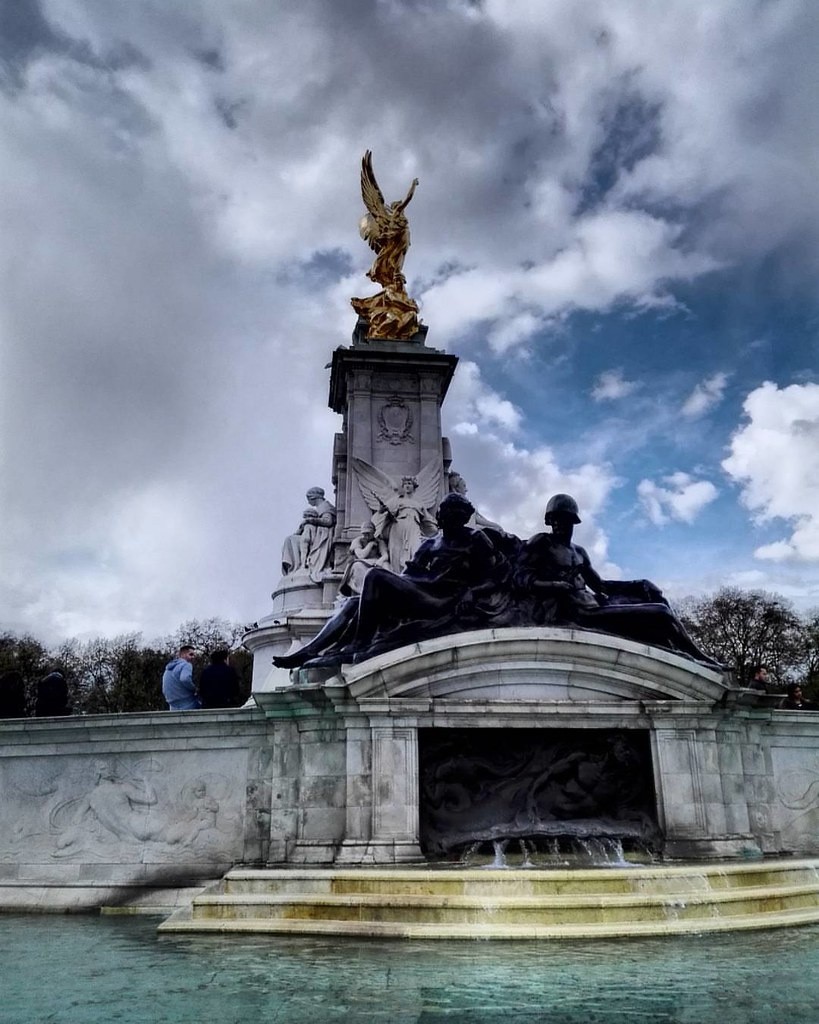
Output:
[273,492,514,669]
[35,668,73,717]
[456,478,504,533]
[509,494,726,666]
[159,645,201,712]
[0,672,26,718]
[427,736,640,834]
[387,476,424,570]
[73,756,219,844]
[743,664,780,695]
[280,486,336,574]
[193,644,247,708]
[781,683,814,709]
[391,178,419,281]
[338,522,389,598]
